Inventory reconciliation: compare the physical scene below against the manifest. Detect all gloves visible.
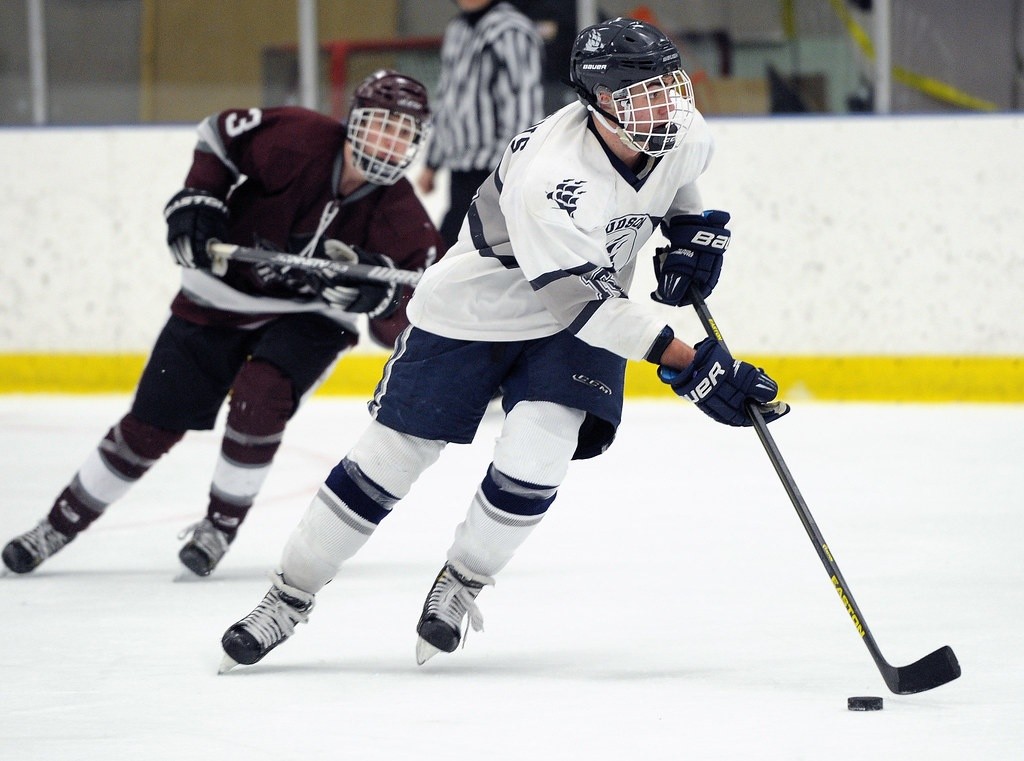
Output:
[656,339,790,427]
[164,190,230,269]
[320,241,398,321]
[650,209,731,307]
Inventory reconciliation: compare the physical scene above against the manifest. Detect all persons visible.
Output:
[0,71,443,580]
[217,17,789,678]
[423,1,660,256]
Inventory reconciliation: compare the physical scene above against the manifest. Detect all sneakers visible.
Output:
[1,518,70,578]
[217,573,317,674]
[415,565,484,665]
[179,519,228,577]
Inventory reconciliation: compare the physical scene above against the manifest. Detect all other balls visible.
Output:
[847,696,883,712]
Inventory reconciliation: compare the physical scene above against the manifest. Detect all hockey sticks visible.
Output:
[685,281,961,695]
[210,242,423,286]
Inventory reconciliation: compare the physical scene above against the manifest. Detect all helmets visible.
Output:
[571,16,695,158]
[346,69,434,186]
